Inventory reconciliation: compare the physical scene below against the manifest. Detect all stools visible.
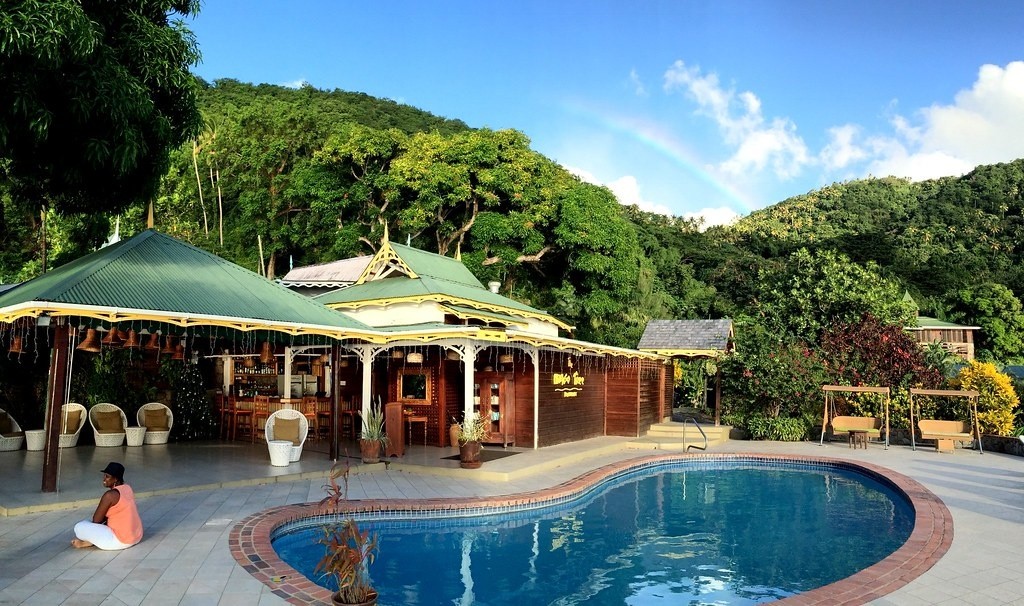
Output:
[936,439,956,453]
[849,429,869,449]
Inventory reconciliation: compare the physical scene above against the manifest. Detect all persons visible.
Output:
[72,462,143,551]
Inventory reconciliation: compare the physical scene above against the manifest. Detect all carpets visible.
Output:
[439,449,523,463]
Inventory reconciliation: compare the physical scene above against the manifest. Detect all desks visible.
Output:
[381,402,405,458]
[25,430,46,451]
[268,440,293,467]
[404,416,429,449]
[125,427,147,447]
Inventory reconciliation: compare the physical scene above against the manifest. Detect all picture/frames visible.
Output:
[397,370,432,406]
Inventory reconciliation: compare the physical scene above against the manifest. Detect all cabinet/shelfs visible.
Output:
[474,368,515,448]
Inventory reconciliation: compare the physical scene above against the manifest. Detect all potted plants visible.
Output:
[356,394,393,464]
[452,410,491,469]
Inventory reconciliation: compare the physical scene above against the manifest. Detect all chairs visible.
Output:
[136,403,174,444]
[58,403,87,447]
[89,403,128,447]
[214,393,272,444]
[0,408,25,452]
[302,395,362,442]
[265,409,309,462]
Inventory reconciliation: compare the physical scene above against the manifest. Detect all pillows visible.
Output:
[67,410,82,431]
[145,408,170,427]
[95,410,124,429]
[273,416,300,440]
[0,412,12,433]
